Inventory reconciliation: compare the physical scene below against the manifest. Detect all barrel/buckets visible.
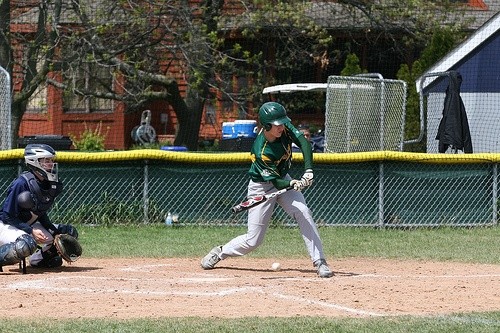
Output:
[222,121,236,138]
[232,120,258,138]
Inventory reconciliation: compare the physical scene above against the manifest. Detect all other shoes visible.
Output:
[32,257,63,269]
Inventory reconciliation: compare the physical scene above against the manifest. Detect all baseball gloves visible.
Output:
[54,234,82,262]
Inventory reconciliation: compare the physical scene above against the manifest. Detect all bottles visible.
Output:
[165,211,174,228]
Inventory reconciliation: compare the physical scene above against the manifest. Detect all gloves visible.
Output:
[302,172,314,187]
[289,179,304,192]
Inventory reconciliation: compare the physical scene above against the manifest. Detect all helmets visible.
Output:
[25,144,58,185]
[258,102,292,131]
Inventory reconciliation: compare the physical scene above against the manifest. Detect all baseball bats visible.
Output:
[230,186,293,214]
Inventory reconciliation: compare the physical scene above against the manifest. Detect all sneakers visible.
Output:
[316,264,334,277]
[201,246,224,269]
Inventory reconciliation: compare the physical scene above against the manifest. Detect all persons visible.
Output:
[201,101,334,279]
[0,143,78,268]
[292,125,322,152]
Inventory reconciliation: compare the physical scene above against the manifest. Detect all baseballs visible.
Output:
[271,262,281,272]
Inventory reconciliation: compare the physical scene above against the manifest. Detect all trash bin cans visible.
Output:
[17,134,73,151]
[234,119,258,139]
[221,122,235,140]
